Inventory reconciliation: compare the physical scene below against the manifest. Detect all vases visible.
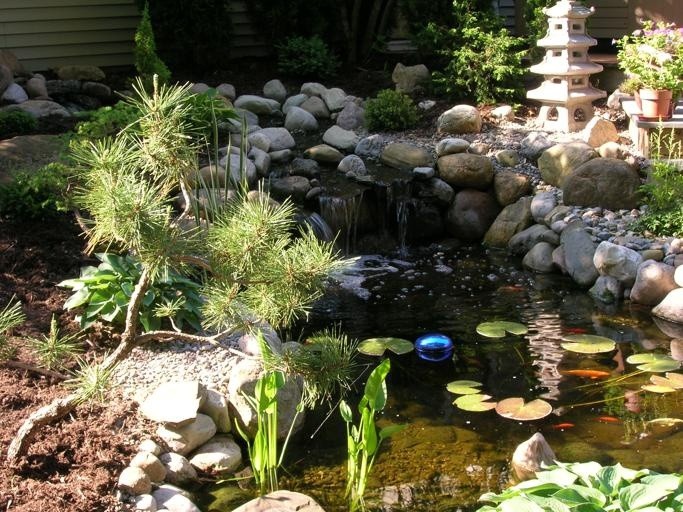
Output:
[639,89,674,118]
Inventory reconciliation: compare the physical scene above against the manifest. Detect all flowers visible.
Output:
[610,18,683,94]
